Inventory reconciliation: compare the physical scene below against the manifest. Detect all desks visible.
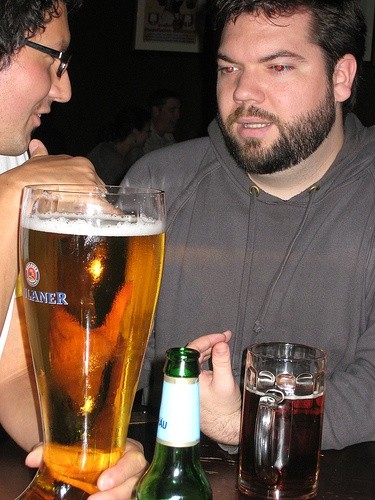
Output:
[0,418,375,500]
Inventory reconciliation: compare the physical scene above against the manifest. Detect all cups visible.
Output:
[237,341,328,500]
[14,183,166,500]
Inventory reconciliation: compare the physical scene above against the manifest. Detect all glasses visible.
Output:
[17,37,72,80]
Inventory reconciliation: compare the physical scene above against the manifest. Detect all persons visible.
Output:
[110,0,375,500]
[138,88,182,156]
[85,106,151,204]
[1,0,152,500]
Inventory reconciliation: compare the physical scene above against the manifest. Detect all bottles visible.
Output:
[134,347,213,500]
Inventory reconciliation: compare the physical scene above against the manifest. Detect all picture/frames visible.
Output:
[135,0,203,53]
